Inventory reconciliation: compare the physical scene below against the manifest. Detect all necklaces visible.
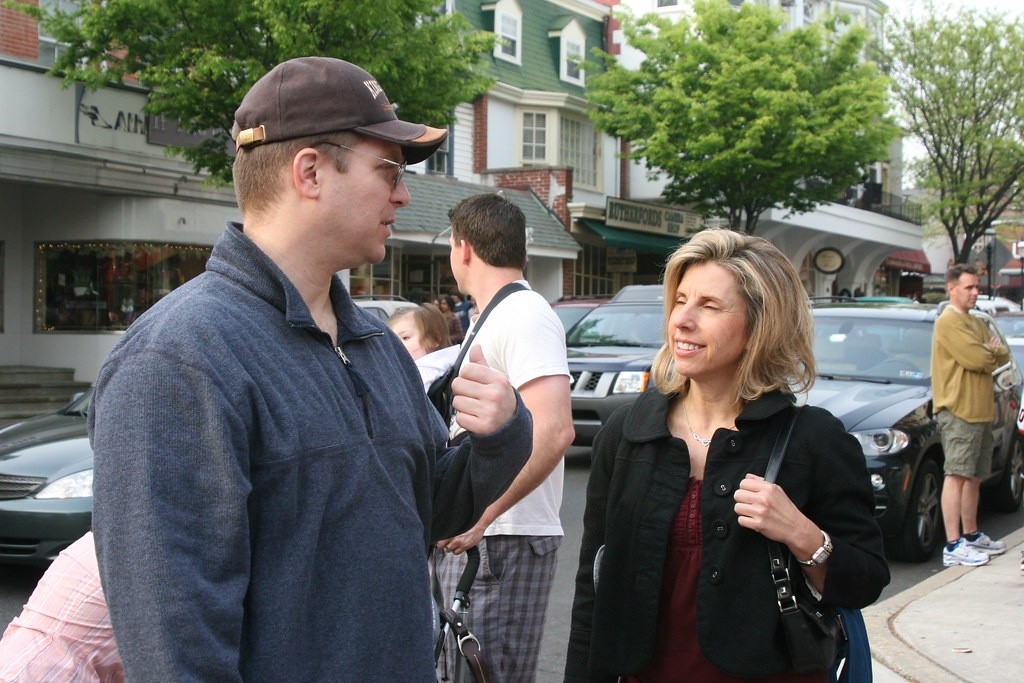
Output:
[684,395,736,446]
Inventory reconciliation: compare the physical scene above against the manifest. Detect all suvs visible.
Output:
[350,292,424,324]
[559,285,674,446]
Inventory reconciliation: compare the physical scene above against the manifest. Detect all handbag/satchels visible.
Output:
[763,406,873,683]
[427,367,460,429]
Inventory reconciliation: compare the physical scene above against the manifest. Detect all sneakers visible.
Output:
[960,531,1006,555]
[943,537,988,566]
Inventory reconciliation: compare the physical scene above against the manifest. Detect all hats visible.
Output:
[231,56,448,165]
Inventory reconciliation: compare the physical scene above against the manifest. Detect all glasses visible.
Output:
[309,140,407,189]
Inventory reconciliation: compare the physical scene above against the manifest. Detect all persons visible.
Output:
[836,288,926,304]
[385,193,575,683]
[87,56,534,683]
[932,263,1010,567]
[562,228,890,683]
[1016,391,1024,570]
[0,531,126,683]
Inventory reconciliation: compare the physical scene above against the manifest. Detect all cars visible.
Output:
[1,386,94,582]
[776,298,1023,563]
[545,291,619,332]
[804,292,1024,396]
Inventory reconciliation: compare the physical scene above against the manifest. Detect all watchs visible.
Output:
[797,530,832,567]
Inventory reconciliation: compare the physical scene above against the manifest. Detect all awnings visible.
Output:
[881,247,930,275]
[581,219,691,253]
